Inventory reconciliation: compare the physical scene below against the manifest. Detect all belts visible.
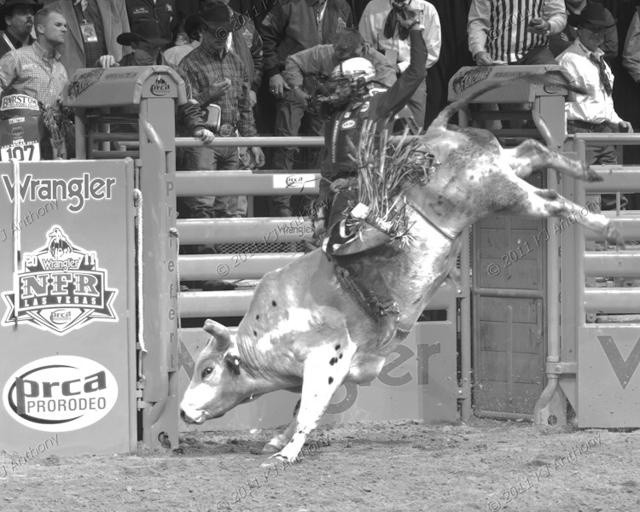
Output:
[568,118,609,132]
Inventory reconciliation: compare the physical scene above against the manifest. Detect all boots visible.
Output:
[351,266,401,349]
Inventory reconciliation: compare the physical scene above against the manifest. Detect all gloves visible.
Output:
[396,1,426,32]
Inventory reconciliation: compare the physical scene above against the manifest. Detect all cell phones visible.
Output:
[528,19,542,27]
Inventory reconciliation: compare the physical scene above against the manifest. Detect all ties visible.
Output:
[591,55,612,97]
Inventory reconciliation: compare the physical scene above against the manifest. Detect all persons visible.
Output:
[1,2,639,289]
[312,0,427,351]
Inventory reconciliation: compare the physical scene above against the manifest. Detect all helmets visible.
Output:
[329,56,376,90]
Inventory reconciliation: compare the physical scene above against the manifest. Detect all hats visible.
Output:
[566,1,619,32]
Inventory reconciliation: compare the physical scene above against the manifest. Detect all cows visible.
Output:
[178,64,629,470]
